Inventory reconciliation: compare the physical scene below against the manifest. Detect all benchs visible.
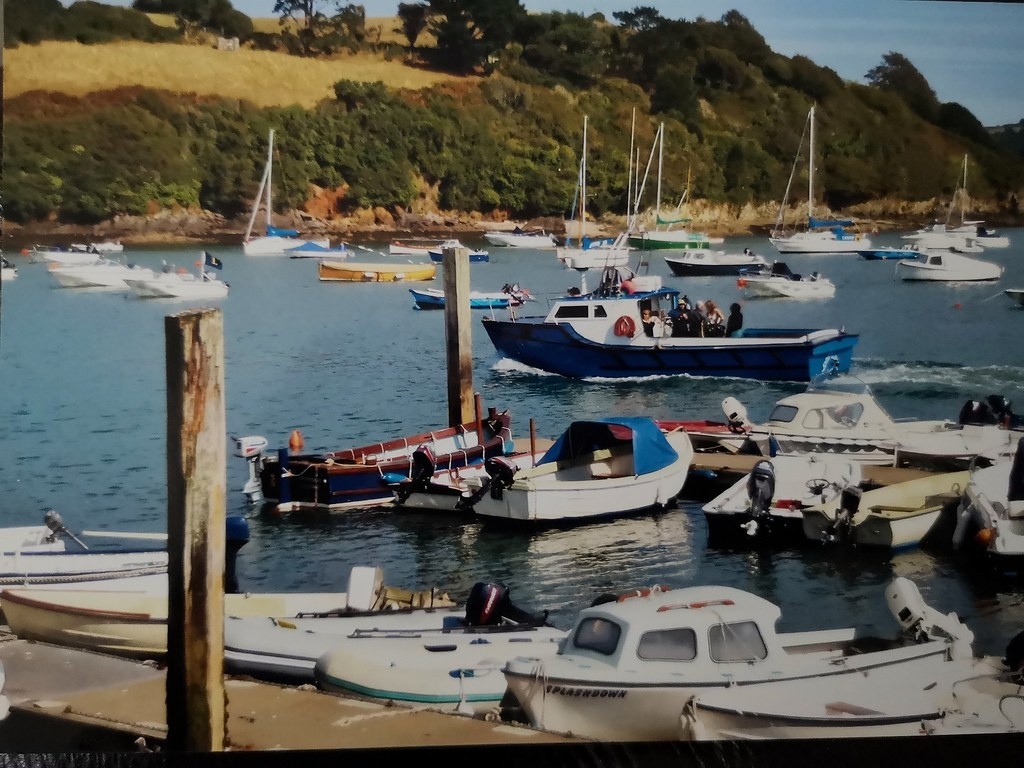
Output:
[868,500,928,512]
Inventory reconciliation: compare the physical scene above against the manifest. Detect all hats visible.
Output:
[678,300,687,305]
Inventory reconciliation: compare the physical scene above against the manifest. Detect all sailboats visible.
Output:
[557,109,640,267]
[242,128,330,255]
[769,107,873,252]
[628,121,725,251]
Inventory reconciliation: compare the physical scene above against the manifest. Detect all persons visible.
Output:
[641,298,724,338]
[725,302,743,337]
[744,248,752,256]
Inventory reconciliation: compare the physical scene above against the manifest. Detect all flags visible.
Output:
[205,253,223,270]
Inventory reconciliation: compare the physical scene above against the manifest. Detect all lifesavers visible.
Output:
[854,235,861,241]
[615,316,636,337]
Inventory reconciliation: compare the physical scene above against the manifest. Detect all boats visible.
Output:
[21,237,124,265]
[700,451,1024,555]
[899,153,1011,251]
[899,249,1001,280]
[389,236,465,255]
[858,247,920,261]
[0,252,19,281]
[408,285,524,309]
[223,566,574,717]
[234,407,512,511]
[0,510,251,652]
[392,426,693,520]
[1004,289,1024,307]
[653,389,1024,470]
[483,229,558,249]
[283,240,348,258]
[428,249,490,265]
[47,260,229,299]
[664,248,771,276]
[482,275,858,384]
[500,575,1024,741]
[318,260,437,282]
